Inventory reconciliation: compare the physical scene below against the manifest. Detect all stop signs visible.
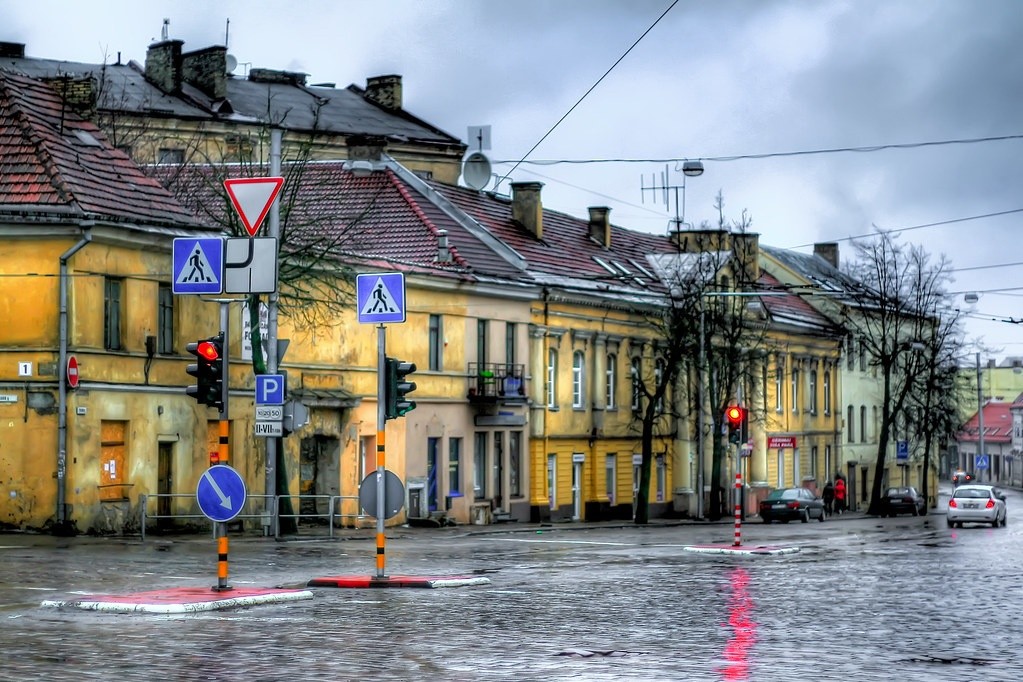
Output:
[65,354,82,387]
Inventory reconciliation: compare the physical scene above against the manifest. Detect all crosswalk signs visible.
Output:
[355,272,408,325]
[171,236,226,295]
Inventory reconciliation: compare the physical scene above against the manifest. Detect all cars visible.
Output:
[759,487,828,523]
[952,468,971,486]
[947,485,1009,528]
[880,486,925,518]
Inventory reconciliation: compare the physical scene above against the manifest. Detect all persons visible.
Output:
[821,480,834,518]
[833,478,847,516]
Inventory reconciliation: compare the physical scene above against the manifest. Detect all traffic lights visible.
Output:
[727,406,742,445]
[195,336,225,414]
[209,450,220,467]
[388,359,418,420]
